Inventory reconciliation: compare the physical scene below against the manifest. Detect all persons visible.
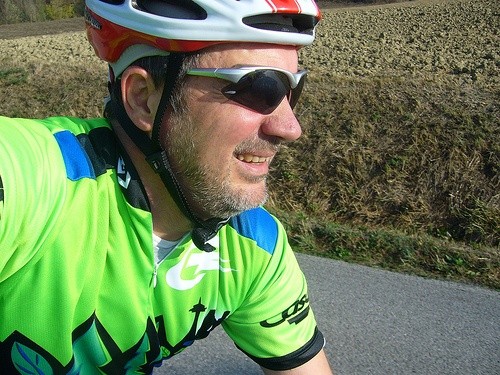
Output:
[0,0,332,375]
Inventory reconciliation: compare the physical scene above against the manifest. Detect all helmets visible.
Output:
[84,0,321,84]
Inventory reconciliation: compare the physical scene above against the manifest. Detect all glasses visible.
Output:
[184,65,307,115]
